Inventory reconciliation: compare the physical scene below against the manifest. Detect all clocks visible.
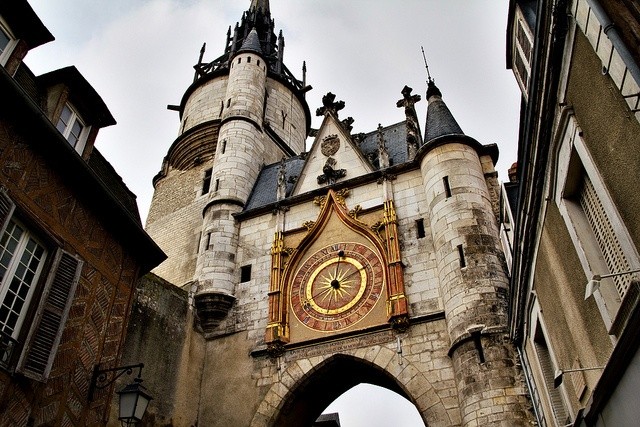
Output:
[264,190,409,347]
